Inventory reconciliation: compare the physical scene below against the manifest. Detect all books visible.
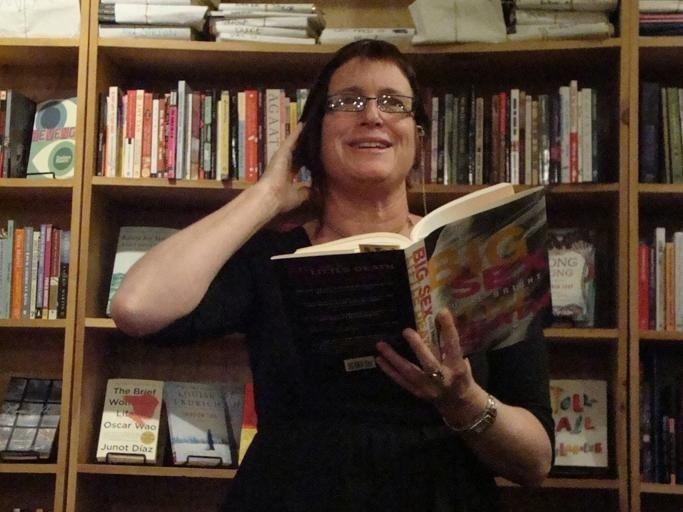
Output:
[97,80,310,178]
[270,182,554,393]
[0,88,77,179]
[639,346,683,484]
[549,379,608,476]
[209,3,327,49]
[0,379,62,463]
[97,379,163,464]
[96,1,209,42]
[639,1,683,36]
[105,226,182,317]
[415,80,598,186]
[505,0,620,40]
[637,227,682,330]
[319,27,415,45]
[544,227,596,328]
[163,381,257,466]
[408,1,507,44]
[0,219,70,320]
[639,80,683,183]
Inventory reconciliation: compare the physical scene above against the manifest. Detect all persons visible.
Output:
[109,38,555,512]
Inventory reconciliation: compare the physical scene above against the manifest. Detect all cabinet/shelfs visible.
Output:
[628,0,683,512]
[67,1,630,512]
[1,0,90,510]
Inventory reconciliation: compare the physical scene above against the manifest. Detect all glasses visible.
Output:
[323,93,416,114]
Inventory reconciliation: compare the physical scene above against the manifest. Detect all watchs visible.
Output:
[443,394,497,436]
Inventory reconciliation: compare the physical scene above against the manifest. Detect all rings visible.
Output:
[425,364,449,385]
